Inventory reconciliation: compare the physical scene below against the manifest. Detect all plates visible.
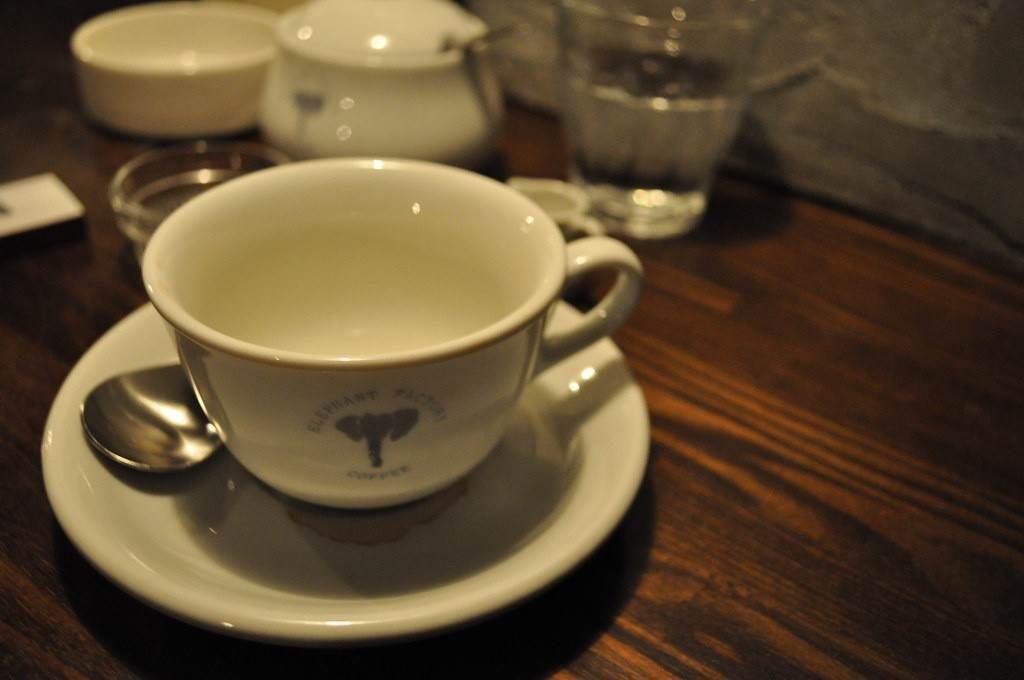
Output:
[36,298,652,644]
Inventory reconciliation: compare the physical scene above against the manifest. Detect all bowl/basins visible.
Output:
[255,0,506,174]
[68,0,284,140]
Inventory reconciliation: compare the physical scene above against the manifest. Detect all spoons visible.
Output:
[79,360,227,477]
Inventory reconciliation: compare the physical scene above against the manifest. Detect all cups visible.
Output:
[558,0,761,239]
[504,178,604,295]
[139,155,644,517]
